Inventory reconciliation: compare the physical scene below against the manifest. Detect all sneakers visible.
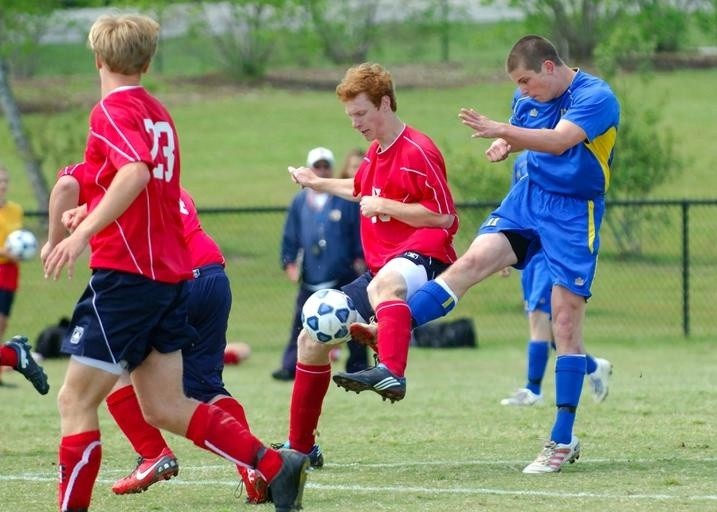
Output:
[6,335,49,395]
[352,320,379,352]
[523,435,580,474]
[272,369,294,381]
[588,358,613,403]
[111,447,178,494]
[332,362,406,403]
[501,387,542,408]
[236,440,323,512]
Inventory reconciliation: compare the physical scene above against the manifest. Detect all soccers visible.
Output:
[6,231,36,260]
[302,289,357,345]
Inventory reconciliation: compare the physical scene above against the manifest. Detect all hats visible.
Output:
[308,148,336,169]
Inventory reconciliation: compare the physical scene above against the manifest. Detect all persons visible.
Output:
[267,33,621,474]
[0,14,308,512]
